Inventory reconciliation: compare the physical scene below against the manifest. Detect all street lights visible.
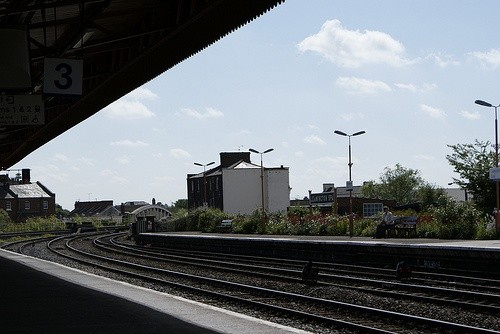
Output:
[473,99,500,238]
[334,130,367,236]
[248,147,274,213]
[192,162,216,217]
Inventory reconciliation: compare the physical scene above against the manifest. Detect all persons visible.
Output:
[376,207,394,239]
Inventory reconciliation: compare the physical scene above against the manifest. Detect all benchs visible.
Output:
[385,214,419,237]
